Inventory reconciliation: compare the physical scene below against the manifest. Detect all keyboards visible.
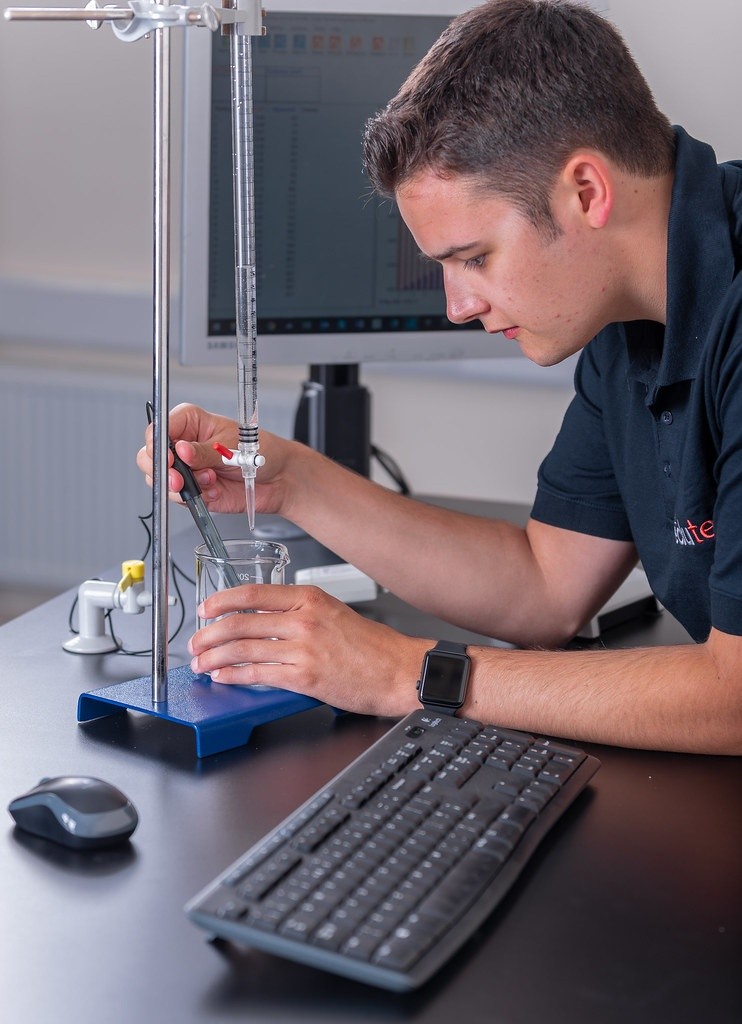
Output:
[183,708,603,995]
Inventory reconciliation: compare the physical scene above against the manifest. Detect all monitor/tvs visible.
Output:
[177,0,529,482]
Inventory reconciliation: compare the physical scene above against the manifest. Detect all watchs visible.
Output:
[419,635,475,716]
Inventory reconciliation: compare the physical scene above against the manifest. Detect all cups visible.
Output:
[194,538,293,682]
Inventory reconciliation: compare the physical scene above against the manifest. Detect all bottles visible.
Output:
[300,358,372,489]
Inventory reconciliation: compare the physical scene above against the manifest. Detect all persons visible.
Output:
[135,0,742,758]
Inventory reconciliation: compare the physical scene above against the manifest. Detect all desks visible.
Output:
[0,493,742,1024]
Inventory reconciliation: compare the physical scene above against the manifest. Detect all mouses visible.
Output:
[7,773,139,852]
